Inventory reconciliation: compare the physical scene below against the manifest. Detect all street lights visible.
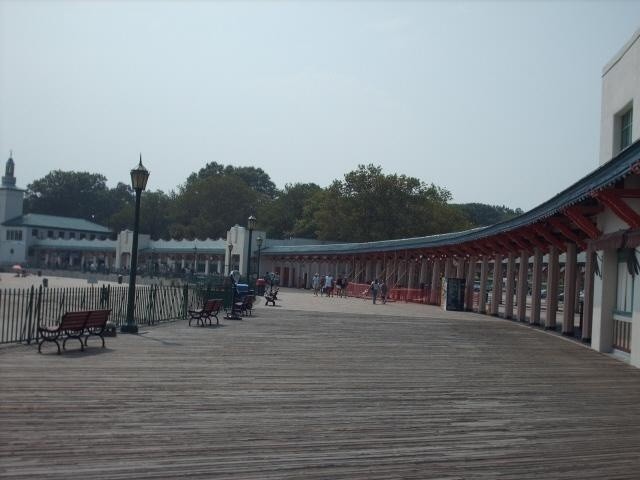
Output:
[246,215,256,284]
[228,242,233,275]
[150,244,197,277]
[127,153,149,321]
[256,235,263,279]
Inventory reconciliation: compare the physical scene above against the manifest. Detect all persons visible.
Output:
[311,272,348,299]
[368,279,380,304]
[379,278,387,304]
[89,263,97,273]
[229,264,241,284]
[263,271,280,292]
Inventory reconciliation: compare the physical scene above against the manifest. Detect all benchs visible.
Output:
[38,309,112,355]
[235,288,279,317]
[189,299,224,326]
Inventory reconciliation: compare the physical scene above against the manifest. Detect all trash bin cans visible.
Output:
[256,278,265,296]
[43,279,48,287]
[446,278,466,311]
[38,270,41,276]
[118,274,122,284]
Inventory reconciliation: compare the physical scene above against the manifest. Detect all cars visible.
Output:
[473,277,584,303]
